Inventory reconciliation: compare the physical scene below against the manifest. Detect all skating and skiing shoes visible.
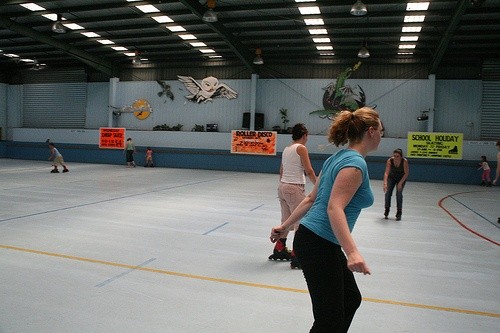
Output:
[395,208,402,220]
[384,207,390,218]
[291,249,302,269]
[268,237,292,260]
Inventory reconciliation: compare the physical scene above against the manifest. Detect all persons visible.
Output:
[383,148,408,221]
[492,137,500,223]
[48,142,68,173]
[123,138,136,168]
[270,107,384,333]
[268,123,317,270]
[478,156,493,187]
[144,147,154,167]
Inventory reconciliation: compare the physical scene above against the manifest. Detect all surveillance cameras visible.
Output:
[417,110,429,121]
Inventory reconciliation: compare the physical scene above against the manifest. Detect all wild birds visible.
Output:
[156,80,174,102]
